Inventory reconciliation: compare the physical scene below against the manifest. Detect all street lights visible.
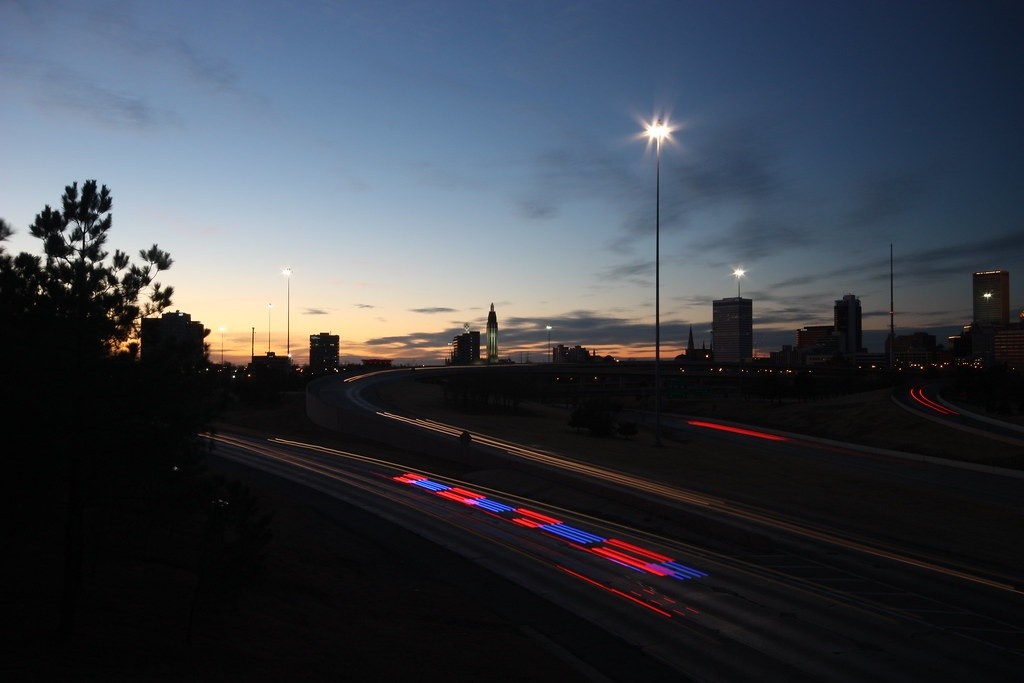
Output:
[651,122,668,446]
[547,324,551,363]
[285,267,291,358]
